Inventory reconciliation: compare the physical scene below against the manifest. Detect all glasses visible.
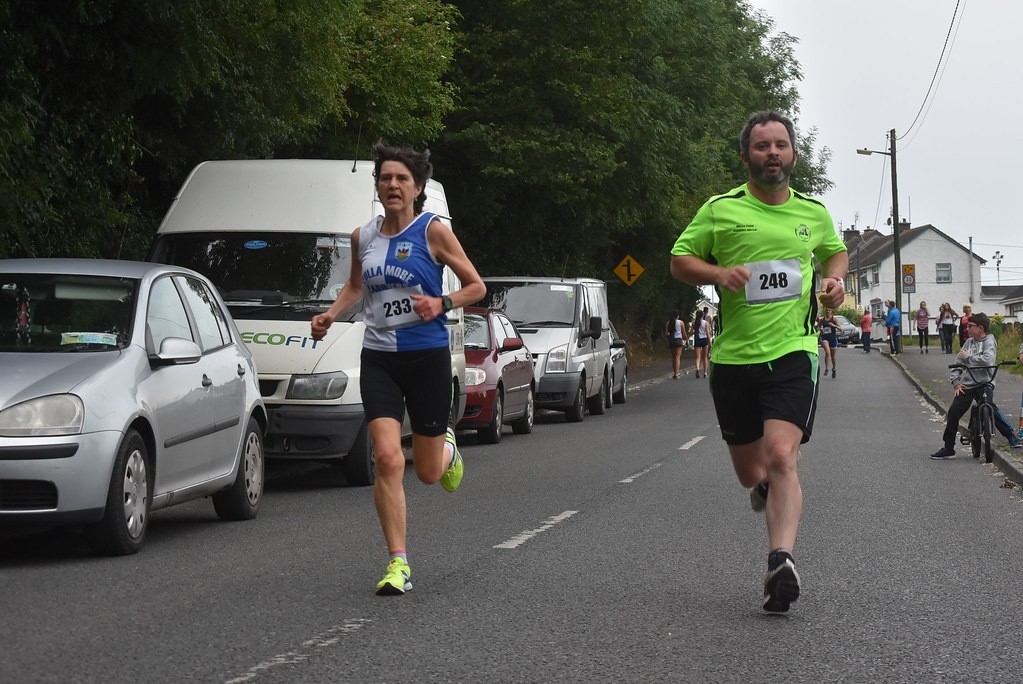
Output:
[968,324,976,328]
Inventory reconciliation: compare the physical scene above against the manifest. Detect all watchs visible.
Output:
[822,275,845,293]
[439,296,453,313]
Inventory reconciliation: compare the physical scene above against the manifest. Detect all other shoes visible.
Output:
[674,374,680,378]
[831,368,837,379]
[925,349,928,354]
[704,373,707,378]
[824,370,829,377]
[862,350,869,354]
[696,370,700,378]
[941,349,952,354]
[920,350,923,354]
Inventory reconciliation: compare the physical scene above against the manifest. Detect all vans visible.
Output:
[478,276,611,422]
[149,160,467,486]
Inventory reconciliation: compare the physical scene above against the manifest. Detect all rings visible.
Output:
[419,312,425,319]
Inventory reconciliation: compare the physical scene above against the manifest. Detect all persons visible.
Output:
[667,112,850,612]
[913,301,930,354]
[929,313,1023,459]
[1017,342,1023,440]
[310,144,487,596]
[664,306,720,378]
[957,303,976,348]
[859,310,872,354]
[935,303,959,354]
[884,299,901,357]
[819,307,842,380]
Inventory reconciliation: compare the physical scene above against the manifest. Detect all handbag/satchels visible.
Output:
[952,323,957,333]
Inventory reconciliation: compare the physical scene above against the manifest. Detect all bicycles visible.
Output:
[949,361,1018,464]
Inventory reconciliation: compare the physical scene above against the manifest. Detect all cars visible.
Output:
[816,316,860,345]
[0,256,269,552]
[608,318,628,406]
[462,305,536,443]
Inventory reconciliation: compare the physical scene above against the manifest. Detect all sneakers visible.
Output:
[441,427,464,492]
[929,447,956,459]
[376,557,415,595]
[761,557,801,615]
[750,482,770,513]
[1010,440,1022,447]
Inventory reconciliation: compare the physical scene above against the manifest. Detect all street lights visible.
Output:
[992,251,1004,286]
[856,149,904,353]
